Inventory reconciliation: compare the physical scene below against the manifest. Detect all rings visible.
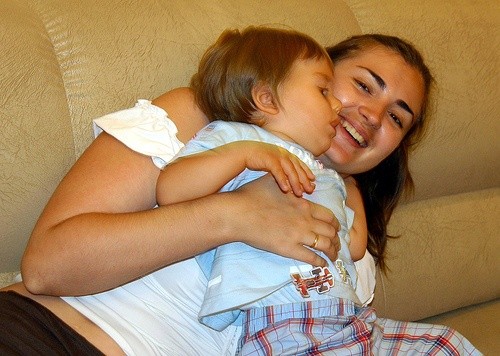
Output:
[310,231,320,249]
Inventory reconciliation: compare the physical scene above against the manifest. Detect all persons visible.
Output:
[156,21,490,356]
[0,33,436,356]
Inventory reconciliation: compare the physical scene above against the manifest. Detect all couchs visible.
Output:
[0,0,500,356]
